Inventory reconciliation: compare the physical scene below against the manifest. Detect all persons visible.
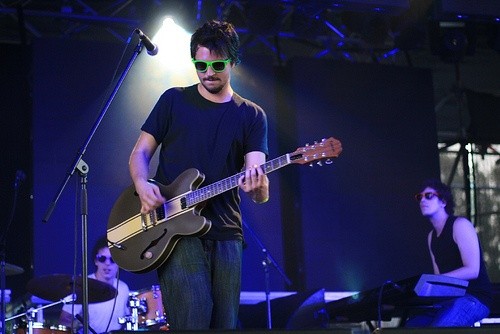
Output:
[59,235,130,334]
[405,179,492,329]
[127,20,270,334]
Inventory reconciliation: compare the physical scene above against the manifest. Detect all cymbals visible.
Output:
[0,261,24,276]
[19,275,118,305]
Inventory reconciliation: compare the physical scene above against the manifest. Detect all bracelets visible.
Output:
[251,196,269,204]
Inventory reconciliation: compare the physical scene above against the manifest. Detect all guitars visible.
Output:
[106,137,343,274]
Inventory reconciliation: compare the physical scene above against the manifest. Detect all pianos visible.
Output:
[323,274,470,334]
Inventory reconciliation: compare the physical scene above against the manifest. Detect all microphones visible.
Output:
[136,28,159,55]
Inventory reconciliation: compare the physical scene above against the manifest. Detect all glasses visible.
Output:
[191,56,232,73]
[95,254,115,264]
[417,191,439,201]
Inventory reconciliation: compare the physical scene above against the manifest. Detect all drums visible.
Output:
[127,286,168,329]
[13,319,75,334]
